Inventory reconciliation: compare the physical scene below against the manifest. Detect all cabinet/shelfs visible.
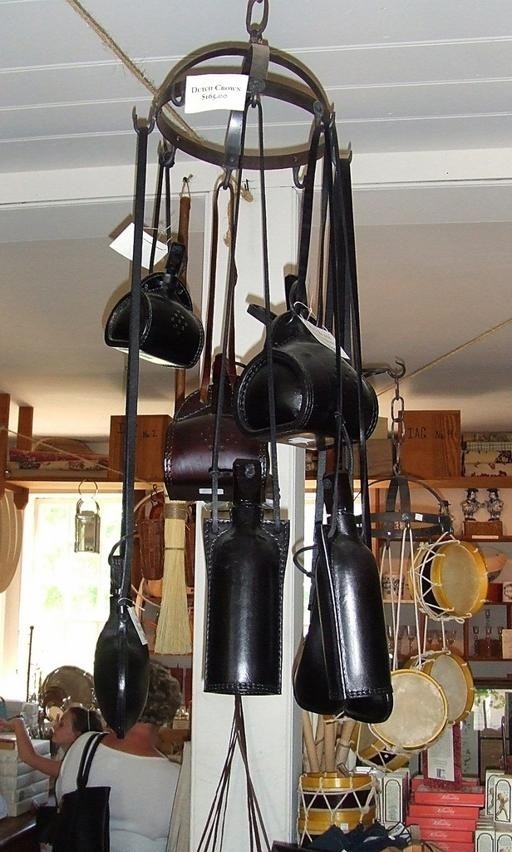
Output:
[369,479,512,662]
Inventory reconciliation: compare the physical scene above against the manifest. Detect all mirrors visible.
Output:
[462,680,512,785]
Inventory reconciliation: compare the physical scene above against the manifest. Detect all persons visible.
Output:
[0,707,103,779]
[54,660,183,852]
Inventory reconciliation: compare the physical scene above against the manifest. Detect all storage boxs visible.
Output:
[351,410,512,478]
[108,415,173,482]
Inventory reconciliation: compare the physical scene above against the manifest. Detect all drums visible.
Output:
[300,772,377,837]
[349,649,474,772]
[411,542,487,622]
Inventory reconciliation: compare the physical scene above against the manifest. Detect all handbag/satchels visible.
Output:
[237,275,379,448]
[52,732,110,852]
[291,470,394,724]
[105,270,205,368]
[203,458,283,696]
[164,355,271,502]
[94,596,150,738]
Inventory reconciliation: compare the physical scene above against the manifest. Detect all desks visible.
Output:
[0,788,59,852]
